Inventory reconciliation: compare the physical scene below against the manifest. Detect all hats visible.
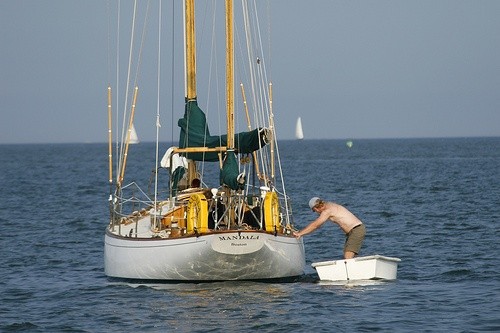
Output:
[308,197,321,208]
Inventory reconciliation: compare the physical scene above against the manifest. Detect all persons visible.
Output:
[292,196,366,260]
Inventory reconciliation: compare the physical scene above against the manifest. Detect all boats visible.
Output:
[310,255,402,282]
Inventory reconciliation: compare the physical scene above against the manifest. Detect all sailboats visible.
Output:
[124,122,141,145]
[104,1,307,281]
